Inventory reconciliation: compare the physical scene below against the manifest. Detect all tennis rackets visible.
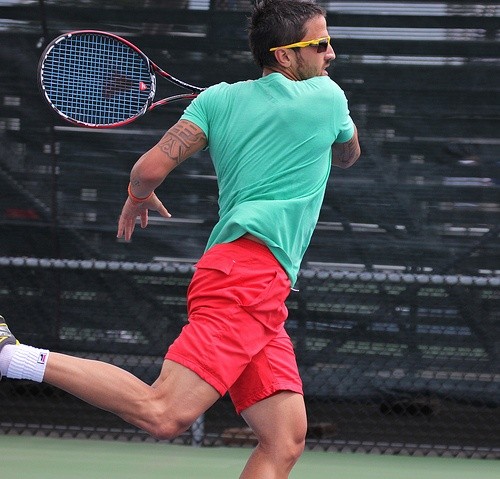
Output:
[36,30,206,129]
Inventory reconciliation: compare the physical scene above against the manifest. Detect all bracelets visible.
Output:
[128,183,153,202]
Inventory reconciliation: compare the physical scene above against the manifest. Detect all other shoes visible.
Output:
[0,315,21,381]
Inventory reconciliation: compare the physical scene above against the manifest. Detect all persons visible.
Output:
[0,0,361,479]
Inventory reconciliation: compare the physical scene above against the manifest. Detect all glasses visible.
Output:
[270,36,331,54]
